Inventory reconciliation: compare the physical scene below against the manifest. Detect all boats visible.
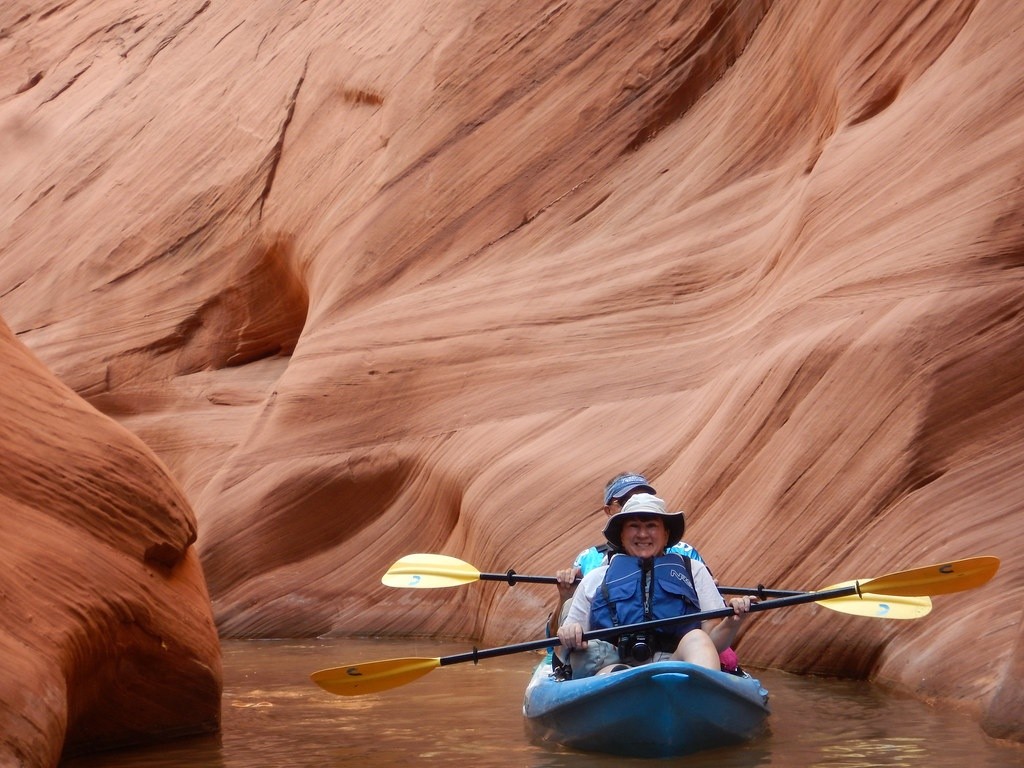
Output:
[518,649,774,759]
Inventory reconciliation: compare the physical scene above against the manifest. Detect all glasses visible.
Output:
[615,491,654,507]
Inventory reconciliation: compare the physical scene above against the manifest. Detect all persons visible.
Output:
[552,492,758,674]
[545,471,763,652]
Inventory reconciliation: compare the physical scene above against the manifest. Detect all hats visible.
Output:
[603,471,657,505]
[601,492,688,548]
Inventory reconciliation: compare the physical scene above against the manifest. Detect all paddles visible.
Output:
[380,552,934,620]
[311,555,1003,698]
